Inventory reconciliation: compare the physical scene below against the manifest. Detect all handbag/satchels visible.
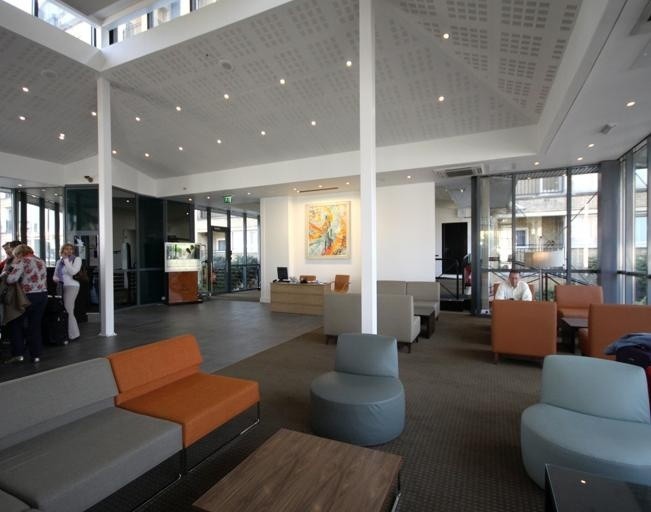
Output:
[74,270,91,288]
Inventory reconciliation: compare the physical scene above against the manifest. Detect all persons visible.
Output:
[53,241,83,344]
[1,243,11,273]
[494,270,532,301]
[6,244,47,366]
[2,240,27,346]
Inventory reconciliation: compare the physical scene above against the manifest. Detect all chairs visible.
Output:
[299,274,316,283]
[491,302,559,364]
[519,356,651,486]
[555,284,604,331]
[310,334,406,449]
[406,282,441,321]
[330,274,351,293]
[494,283,536,302]
[377,280,406,294]
[579,302,651,358]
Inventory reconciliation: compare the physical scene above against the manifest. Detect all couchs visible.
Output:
[323,293,422,354]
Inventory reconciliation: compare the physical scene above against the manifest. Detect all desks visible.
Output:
[269,280,332,315]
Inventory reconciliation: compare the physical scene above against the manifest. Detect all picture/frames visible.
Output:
[304,199,352,262]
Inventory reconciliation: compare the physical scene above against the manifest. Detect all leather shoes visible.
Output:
[4,355,24,365]
[31,357,41,364]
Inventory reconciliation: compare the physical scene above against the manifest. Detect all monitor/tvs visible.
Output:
[165,242,202,272]
[277,267,288,282]
